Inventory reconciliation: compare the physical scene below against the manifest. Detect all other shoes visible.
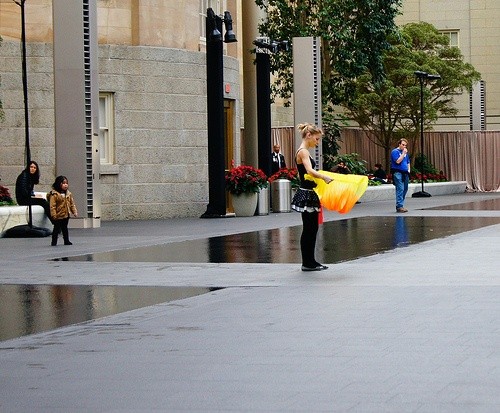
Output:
[397,207,408,212]
[51,241,57,245]
[64,241,72,244]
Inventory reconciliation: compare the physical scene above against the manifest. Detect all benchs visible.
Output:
[0,205,54,237]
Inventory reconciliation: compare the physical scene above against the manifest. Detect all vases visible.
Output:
[231,190,258,216]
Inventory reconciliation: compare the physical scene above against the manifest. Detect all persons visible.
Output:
[330,162,352,174]
[15,161,63,234]
[47,176,78,246]
[391,139,410,213]
[291,123,334,271]
[370,163,389,184]
[271,144,287,169]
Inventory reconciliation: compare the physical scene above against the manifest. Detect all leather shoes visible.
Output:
[301,263,328,270]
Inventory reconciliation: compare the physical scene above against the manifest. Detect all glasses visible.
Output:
[402,144,407,147]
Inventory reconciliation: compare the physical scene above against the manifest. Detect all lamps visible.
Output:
[207,8,289,53]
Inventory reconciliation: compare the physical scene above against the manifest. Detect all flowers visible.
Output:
[225,160,267,195]
[268,167,298,182]
